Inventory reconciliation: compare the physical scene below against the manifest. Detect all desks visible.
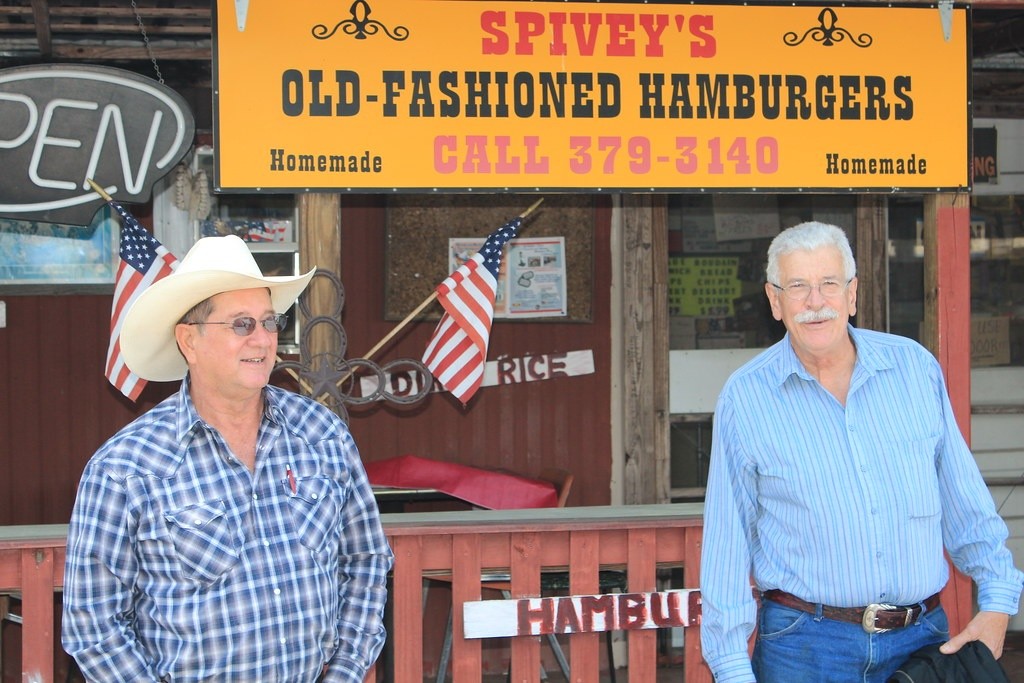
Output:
[370,486,549,680]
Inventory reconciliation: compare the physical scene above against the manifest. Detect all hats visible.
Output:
[119,235,316,381]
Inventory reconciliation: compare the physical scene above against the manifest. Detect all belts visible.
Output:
[763,589,941,634]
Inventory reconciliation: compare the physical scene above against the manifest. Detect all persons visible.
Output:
[700,222,1024,682]
[60,235,394,683]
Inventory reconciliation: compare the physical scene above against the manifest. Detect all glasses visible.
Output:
[189,313,289,337]
[772,280,853,299]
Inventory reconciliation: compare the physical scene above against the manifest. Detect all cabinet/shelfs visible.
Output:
[190,146,303,350]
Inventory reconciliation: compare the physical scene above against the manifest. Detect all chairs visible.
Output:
[423,476,574,683]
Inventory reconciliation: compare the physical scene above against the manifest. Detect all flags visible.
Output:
[105,202,182,402]
[422,218,523,406]
